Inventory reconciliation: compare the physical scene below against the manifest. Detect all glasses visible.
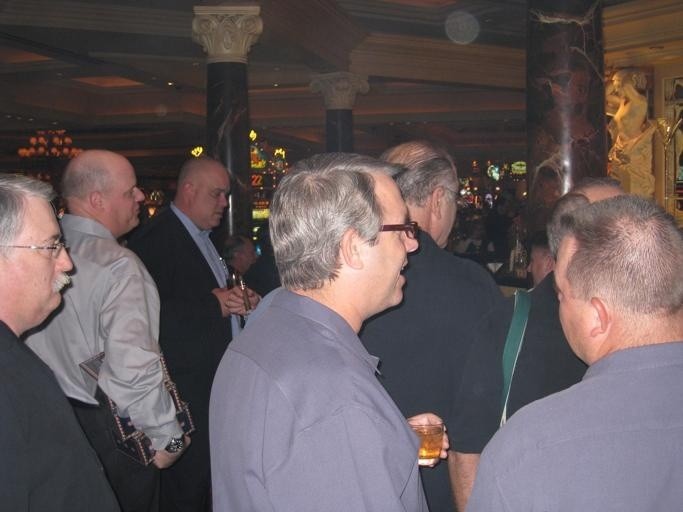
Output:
[368,220,419,241]
[0,242,64,260]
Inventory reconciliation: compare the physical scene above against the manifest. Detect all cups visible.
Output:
[224,272,249,290]
[406,418,444,468]
